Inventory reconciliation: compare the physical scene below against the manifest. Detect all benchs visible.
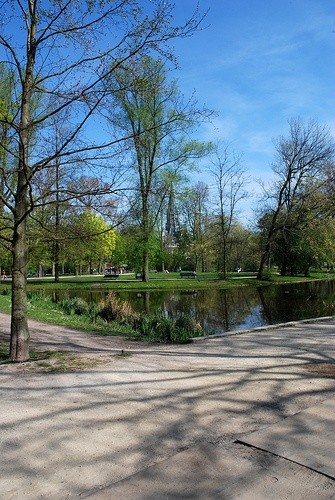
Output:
[180,264,196,279]
